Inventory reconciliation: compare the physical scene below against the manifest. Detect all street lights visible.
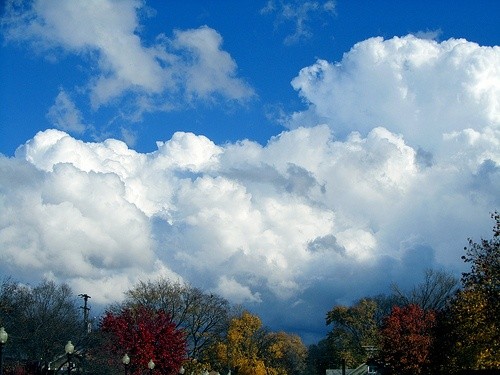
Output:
[147,359,156,375]
[0,326,7,375]
[121,353,130,375]
[64,340,75,375]
